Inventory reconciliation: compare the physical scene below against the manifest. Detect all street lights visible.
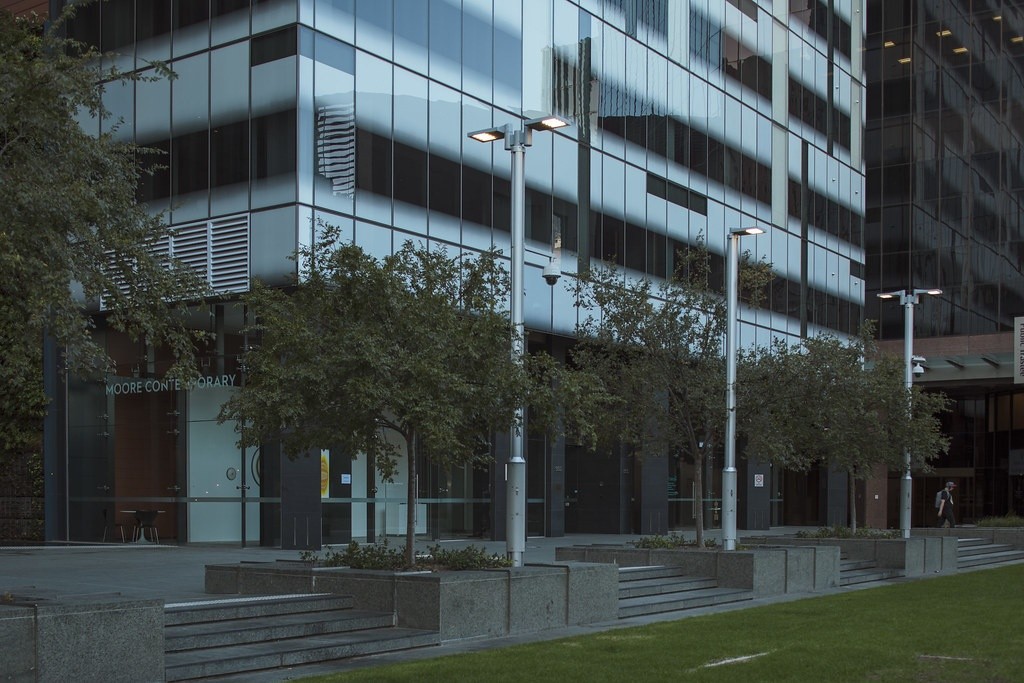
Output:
[722,226,767,551]
[467,115,571,567]
[878,288,942,538]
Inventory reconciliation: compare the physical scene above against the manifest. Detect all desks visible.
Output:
[120,510,166,544]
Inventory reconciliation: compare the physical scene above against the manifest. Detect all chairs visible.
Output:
[135,509,160,545]
[102,509,127,543]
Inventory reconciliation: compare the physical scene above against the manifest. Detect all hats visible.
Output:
[946,482,956,486]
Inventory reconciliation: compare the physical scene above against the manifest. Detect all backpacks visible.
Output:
[935,490,949,508]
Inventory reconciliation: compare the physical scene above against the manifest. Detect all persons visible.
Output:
[938,482,957,528]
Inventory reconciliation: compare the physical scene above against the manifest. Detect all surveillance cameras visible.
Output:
[543,263,562,285]
[912,363,924,377]
